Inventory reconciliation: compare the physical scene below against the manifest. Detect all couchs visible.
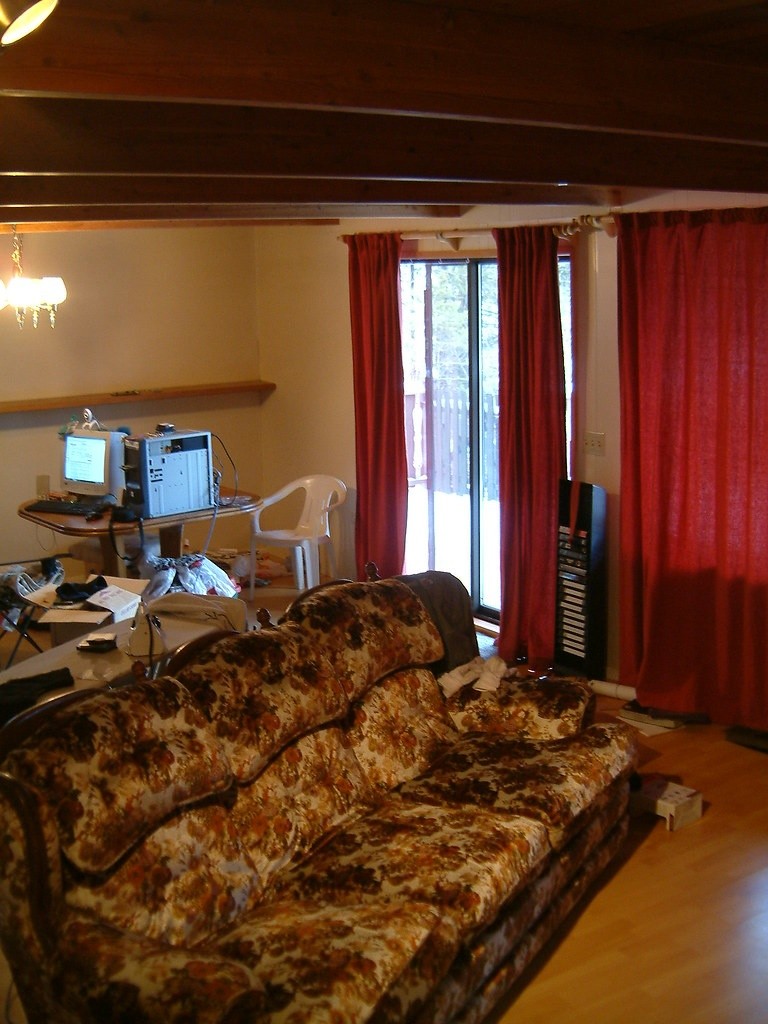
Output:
[0,562,637,1024]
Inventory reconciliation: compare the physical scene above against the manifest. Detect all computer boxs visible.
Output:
[123,428,215,517]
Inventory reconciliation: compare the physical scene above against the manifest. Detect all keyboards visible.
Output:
[24,499,109,516]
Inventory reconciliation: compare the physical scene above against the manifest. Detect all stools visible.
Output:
[0,585,44,670]
[69,532,190,579]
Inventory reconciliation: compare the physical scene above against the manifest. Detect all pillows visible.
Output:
[443,677,595,740]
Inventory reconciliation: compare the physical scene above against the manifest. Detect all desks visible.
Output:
[18,485,264,578]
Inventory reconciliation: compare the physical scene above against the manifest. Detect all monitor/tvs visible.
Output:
[61,429,128,506]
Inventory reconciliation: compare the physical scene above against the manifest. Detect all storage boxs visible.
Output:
[635,778,703,831]
[23,574,150,648]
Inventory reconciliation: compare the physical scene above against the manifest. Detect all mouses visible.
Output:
[86,512,103,521]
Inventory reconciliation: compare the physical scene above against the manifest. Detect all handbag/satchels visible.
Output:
[125,598,163,656]
[109,506,143,560]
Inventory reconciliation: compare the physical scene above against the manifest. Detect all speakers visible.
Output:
[36,475,50,501]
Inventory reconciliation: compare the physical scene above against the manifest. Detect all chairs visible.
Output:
[249,474,347,602]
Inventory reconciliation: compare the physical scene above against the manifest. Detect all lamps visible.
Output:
[0,0,59,48]
[0,225,67,330]
